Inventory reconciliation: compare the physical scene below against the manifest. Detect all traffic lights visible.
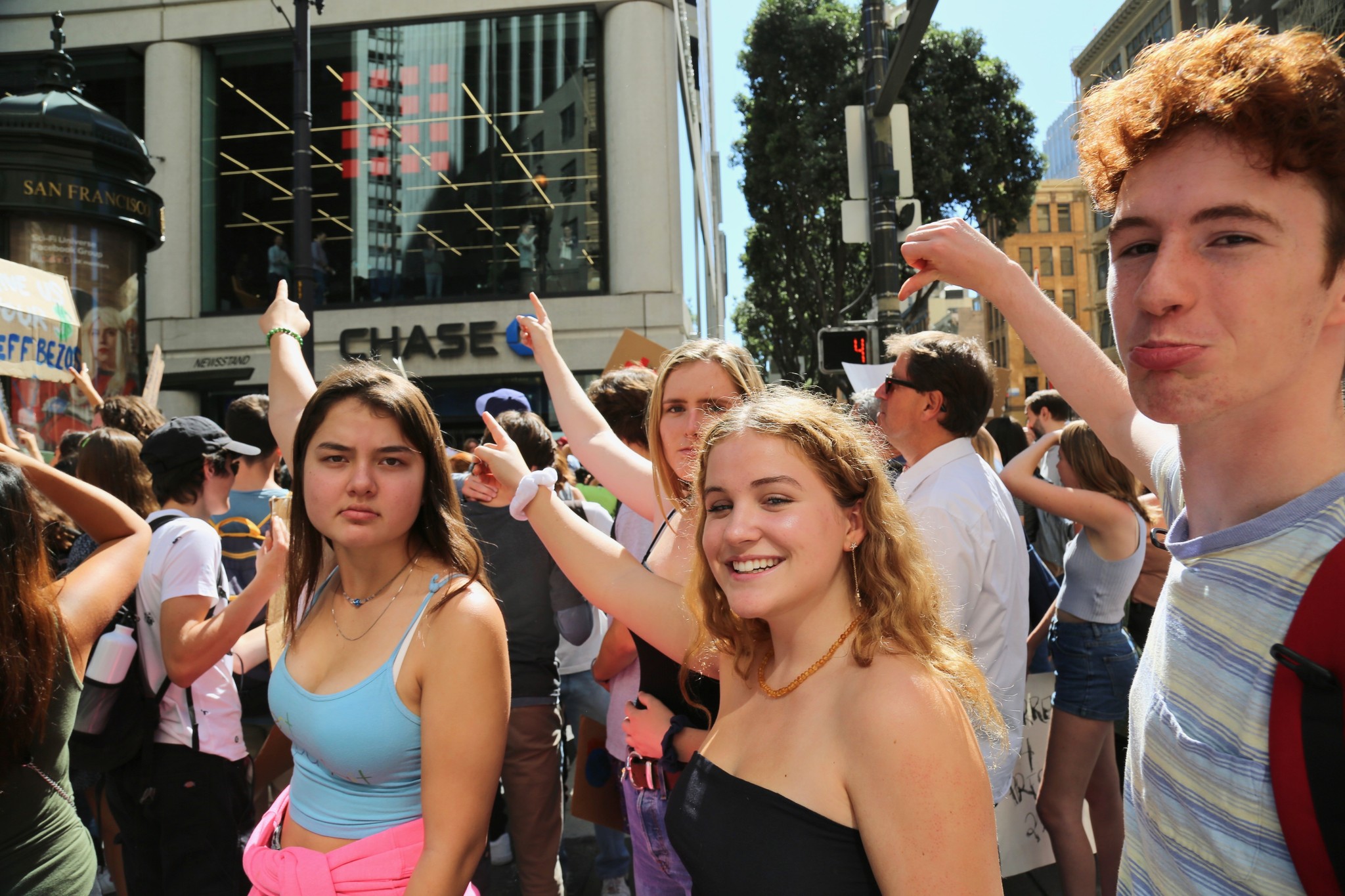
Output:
[817,325,871,374]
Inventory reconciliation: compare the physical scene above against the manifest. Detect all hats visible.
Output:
[476,387,531,421]
[141,415,262,456]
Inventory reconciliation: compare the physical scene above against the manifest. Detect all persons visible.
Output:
[237,279,512,896]
[894,15,1345,896]
[471,386,1004,896]
[0,289,1151,896]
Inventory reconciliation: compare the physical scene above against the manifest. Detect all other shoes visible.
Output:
[602,877,631,895]
[490,834,512,864]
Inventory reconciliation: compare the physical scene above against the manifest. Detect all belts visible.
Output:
[625,746,682,792]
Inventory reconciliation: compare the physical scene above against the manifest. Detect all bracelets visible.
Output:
[508,468,560,523]
[264,326,304,354]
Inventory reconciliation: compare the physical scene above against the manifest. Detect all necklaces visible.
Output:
[340,547,419,609]
[330,547,422,641]
[757,610,871,698]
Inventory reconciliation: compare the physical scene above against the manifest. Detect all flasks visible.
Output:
[73,611,137,736]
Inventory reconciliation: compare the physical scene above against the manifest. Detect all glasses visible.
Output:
[230,460,240,475]
[885,375,920,395]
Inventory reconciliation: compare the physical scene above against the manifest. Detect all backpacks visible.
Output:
[72,516,227,773]
[207,491,293,634]
